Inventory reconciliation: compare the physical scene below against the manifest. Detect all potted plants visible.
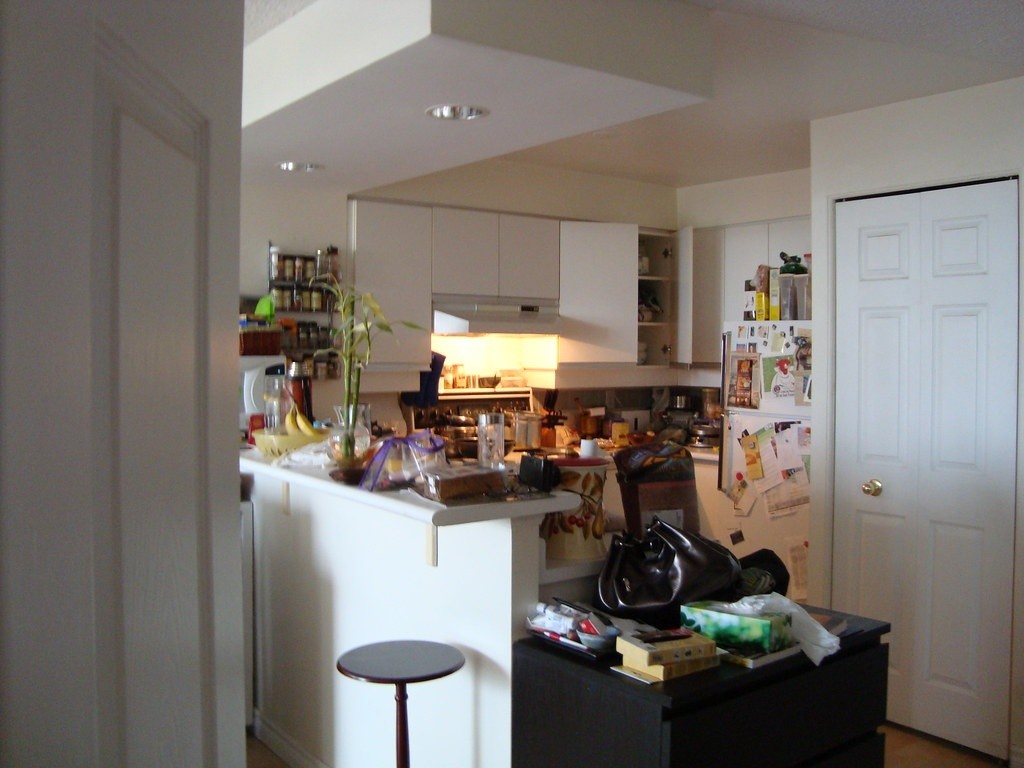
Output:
[307,266,432,469]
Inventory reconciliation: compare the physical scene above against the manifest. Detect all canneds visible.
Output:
[271,256,341,378]
[453,364,465,388]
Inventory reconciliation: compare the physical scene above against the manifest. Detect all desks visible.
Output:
[513,599,895,768]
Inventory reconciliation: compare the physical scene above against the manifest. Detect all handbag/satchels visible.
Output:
[359,428,443,492]
[597,517,742,626]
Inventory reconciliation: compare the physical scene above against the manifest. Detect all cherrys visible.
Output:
[568,502,590,527]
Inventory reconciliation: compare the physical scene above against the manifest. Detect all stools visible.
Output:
[336,639,465,768]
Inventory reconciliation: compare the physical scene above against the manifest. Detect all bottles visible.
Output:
[272,254,329,350]
[779,256,808,321]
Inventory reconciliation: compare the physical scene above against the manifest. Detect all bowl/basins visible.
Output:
[576,626,621,648]
[478,377,501,388]
[455,437,515,459]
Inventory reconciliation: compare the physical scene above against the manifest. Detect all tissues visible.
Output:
[679,590,841,667]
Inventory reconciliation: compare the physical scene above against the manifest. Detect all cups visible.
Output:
[516,412,540,452]
[479,415,505,465]
[265,364,284,432]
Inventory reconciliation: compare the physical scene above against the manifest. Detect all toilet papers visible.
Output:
[580,438,609,459]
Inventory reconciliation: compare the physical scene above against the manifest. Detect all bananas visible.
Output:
[284,401,320,436]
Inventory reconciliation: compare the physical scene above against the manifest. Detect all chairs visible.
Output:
[613,444,701,538]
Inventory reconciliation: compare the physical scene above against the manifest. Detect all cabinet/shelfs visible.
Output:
[768,218,812,269]
[432,207,559,300]
[723,221,773,321]
[683,227,724,363]
[560,220,674,364]
[351,200,432,392]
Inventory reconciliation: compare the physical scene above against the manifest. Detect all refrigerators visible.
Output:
[719,321,813,603]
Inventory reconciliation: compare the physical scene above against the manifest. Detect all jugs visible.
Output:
[286,361,313,425]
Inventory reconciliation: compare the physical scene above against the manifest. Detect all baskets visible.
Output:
[253,426,331,460]
